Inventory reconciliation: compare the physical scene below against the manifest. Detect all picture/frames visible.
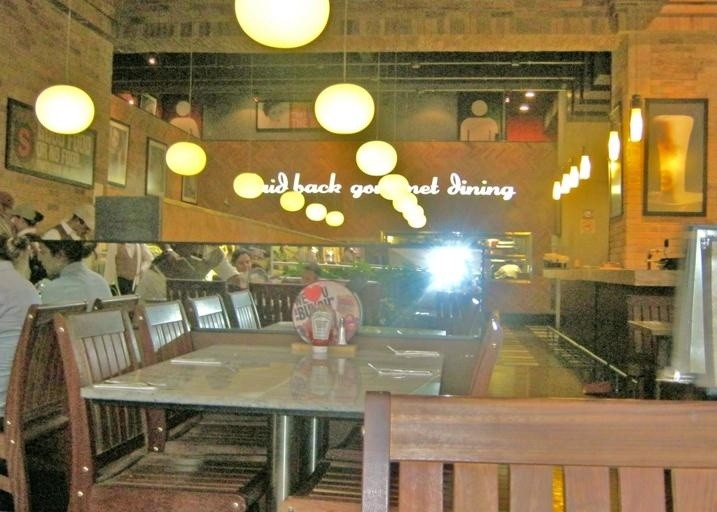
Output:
[106,118,130,188]
[643,97,708,217]
[145,136,167,197]
[181,176,198,205]
[5,96,98,190]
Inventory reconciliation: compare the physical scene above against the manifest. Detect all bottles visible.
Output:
[338,317,347,345]
[309,300,335,345]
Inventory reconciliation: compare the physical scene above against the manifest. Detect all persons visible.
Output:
[0,214,42,435]
[40,202,95,240]
[231,248,252,272]
[36,239,114,313]
[131,241,244,329]
[261,102,284,129]
[302,263,321,284]
[10,203,44,242]
[104,241,155,296]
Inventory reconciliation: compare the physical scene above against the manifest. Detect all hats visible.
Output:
[4,203,36,224]
[72,204,95,232]
[0,191,16,208]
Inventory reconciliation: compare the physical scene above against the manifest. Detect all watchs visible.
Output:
[163,249,173,255]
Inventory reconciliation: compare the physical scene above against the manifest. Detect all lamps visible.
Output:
[233,0,332,51]
[312,0,376,136]
[305,202,328,223]
[377,174,428,230]
[32,0,97,136]
[279,190,306,213]
[230,55,266,201]
[354,52,399,177]
[324,210,345,228]
[552,14,642,200]
[165,53,207,177]
